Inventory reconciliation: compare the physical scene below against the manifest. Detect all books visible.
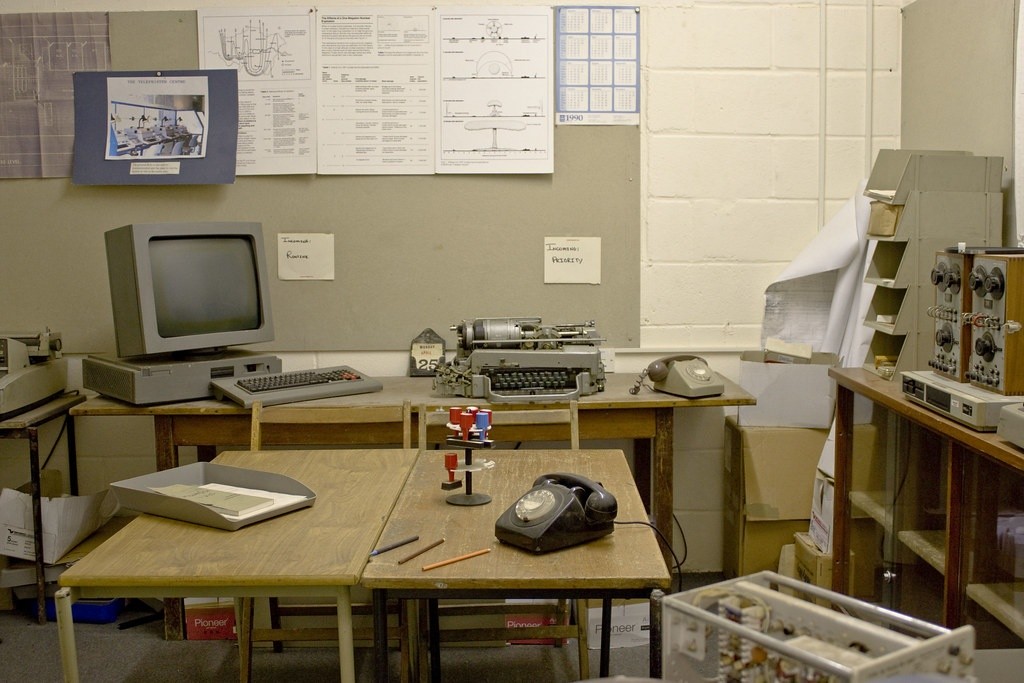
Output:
[147,484,275,516]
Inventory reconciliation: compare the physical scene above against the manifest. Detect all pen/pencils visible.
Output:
[368,534,419,555]
[422,548,491,570]
[397,538,446,564]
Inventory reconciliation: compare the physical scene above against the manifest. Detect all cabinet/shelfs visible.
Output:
[0,387,89,629]
[826,367,1024,648]
[861,147,1007,380]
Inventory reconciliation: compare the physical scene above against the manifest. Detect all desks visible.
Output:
[363,449,670,683]
[49,448,422,683]
[116,128,193,155]
[70,374,761,644]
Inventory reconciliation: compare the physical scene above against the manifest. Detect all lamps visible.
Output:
[137,115,148,128]
[160,115,170,127]
[173,116,183,126]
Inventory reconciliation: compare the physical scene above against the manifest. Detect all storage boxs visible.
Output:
[0,467,117,564]
[725,340,885,609]
[183,597,655,647]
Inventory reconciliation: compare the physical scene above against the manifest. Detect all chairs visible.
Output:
[155,134,201,156]
[418,401,590,683]
[239,399,411,683]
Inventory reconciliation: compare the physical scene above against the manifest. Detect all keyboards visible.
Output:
[210,364,383,408]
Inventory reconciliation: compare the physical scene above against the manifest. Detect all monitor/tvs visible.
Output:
[103,220,274,359]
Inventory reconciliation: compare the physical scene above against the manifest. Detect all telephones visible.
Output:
[646,351,726,397]
[494,471,618,555]
